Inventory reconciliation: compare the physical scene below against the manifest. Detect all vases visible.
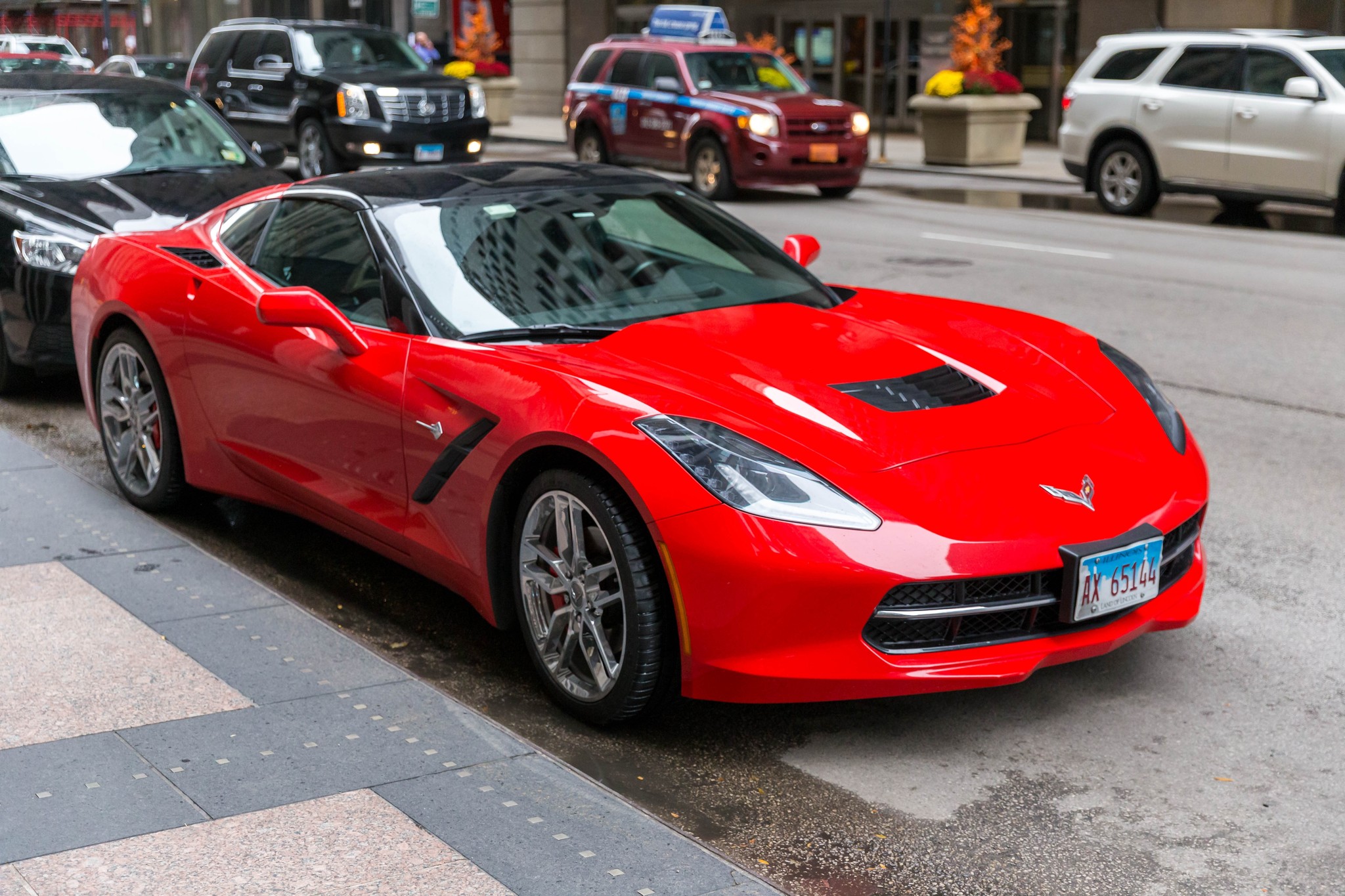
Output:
[907,92,1042,166]
[443,76,520,124]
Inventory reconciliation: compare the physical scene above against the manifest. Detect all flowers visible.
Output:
[444,61,510,80]
[924,69,1023,97]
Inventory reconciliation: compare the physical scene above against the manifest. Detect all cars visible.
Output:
[1,30,196,89]
[0,67,300,401]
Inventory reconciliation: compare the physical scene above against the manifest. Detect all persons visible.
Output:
[411,32,440,70]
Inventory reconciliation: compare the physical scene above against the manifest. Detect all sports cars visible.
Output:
[67,159,1210,733]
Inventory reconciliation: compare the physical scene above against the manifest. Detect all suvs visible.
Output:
[1056,28,1345,232]
[559,2,871,199]
[184,15,492,180]
